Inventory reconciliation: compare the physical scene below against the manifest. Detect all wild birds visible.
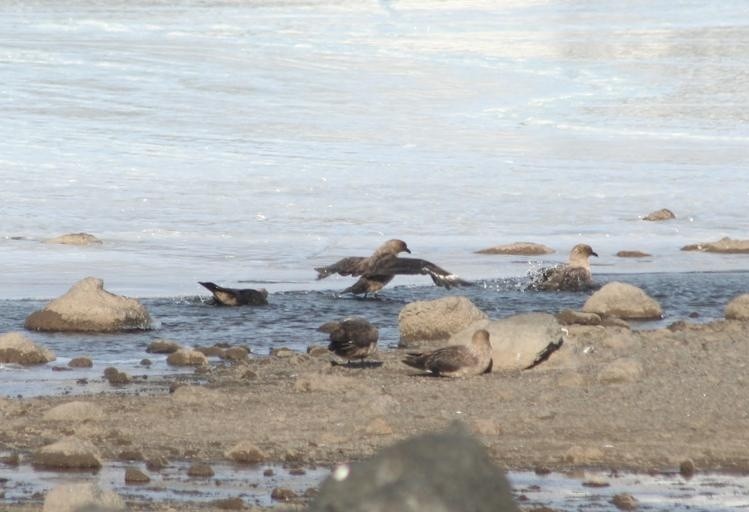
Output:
[198,280,268,307]
[401,328,493,379]
[526,244,601,294]
[317,316,380,368]
[313,239,474,300]
